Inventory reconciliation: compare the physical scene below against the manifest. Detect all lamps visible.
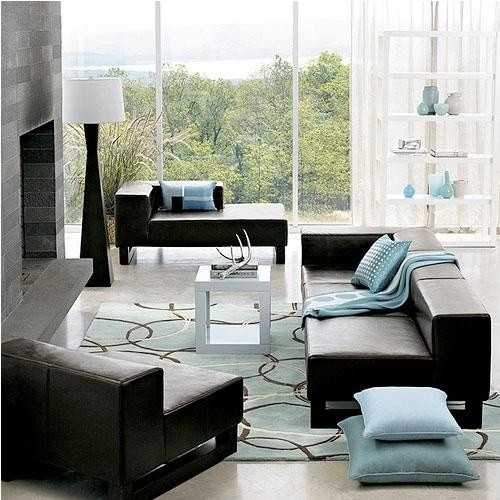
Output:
[58,77,127,287]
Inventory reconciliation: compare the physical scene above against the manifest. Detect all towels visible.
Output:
[300,247,461,331]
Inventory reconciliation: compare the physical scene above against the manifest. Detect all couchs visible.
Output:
[299,226,491,430]
[1,335,243,499]
[115,180,288,265]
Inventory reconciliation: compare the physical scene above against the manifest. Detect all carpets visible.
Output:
[77,301,499,460]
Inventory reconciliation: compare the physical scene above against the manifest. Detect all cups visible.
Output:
[434,104,449,116]
[171,195,184,213]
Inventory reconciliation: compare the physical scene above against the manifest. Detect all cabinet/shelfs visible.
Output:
[380,31,495,228]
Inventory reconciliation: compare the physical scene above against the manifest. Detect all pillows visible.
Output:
[158,181,219,211]
[352,385,464,441]
[351,233,413,292]
[337,414,481,484]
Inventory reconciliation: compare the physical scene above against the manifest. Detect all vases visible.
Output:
[439,170,454,199]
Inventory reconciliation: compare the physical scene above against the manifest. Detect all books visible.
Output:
[430,147,468,158]
[210,260,258,277]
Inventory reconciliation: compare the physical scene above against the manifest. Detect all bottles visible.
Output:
[441,171,454,198]
